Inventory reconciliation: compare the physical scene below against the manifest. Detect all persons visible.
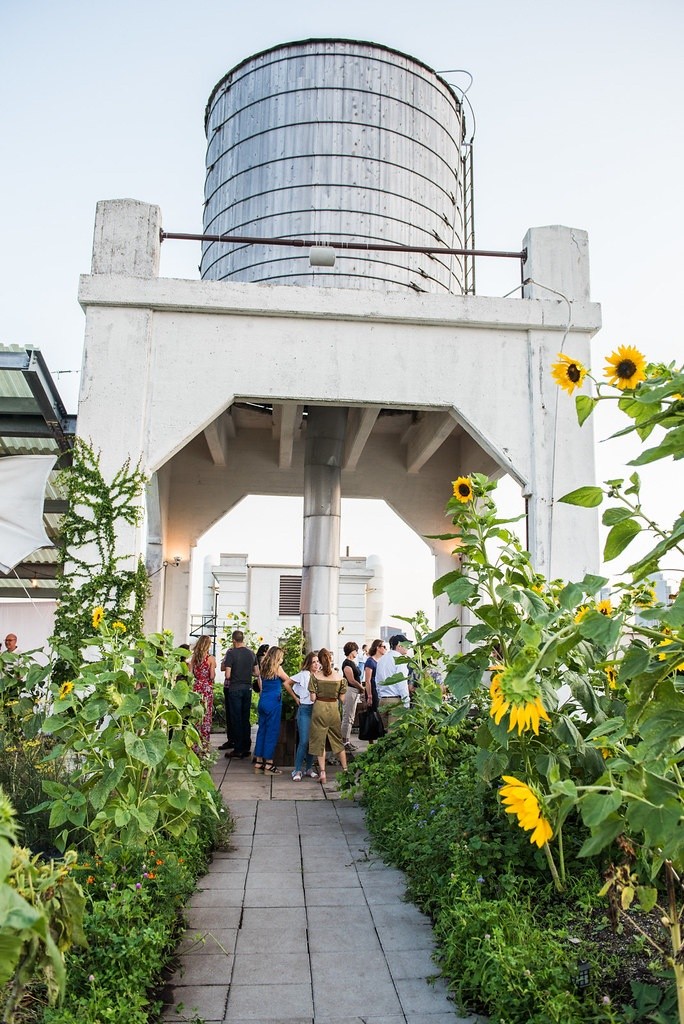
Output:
[281,634,446,784]
[4,634,24,729]
[135,635,216,756]
[491,643,504,660]
[218,631,290,776]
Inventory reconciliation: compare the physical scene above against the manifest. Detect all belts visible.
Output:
[382,695,400,698]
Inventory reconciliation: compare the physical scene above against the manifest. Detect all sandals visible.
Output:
[293,771,302,782]
[304,769,318,778]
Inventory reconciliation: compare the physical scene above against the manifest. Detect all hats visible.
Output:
[389,635,413,645]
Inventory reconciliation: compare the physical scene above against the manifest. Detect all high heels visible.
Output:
[254,762,264,774]
[264,763,282,776]
[318,770,327,784]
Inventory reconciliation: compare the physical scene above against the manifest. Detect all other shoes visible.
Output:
[350,742,359,748]
[345,744,357,751]
[225,751,247,758]
[218,743,234,750]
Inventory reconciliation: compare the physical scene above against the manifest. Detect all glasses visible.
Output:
[5,639,16,642]
[379,646,386,648]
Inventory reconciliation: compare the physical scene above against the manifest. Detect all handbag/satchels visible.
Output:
[358,705,385,741]
[253,680,260,693]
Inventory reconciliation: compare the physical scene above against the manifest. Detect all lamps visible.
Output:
[565,938,593,1003]
[172,557,182,566]
[310,245,336,265]
